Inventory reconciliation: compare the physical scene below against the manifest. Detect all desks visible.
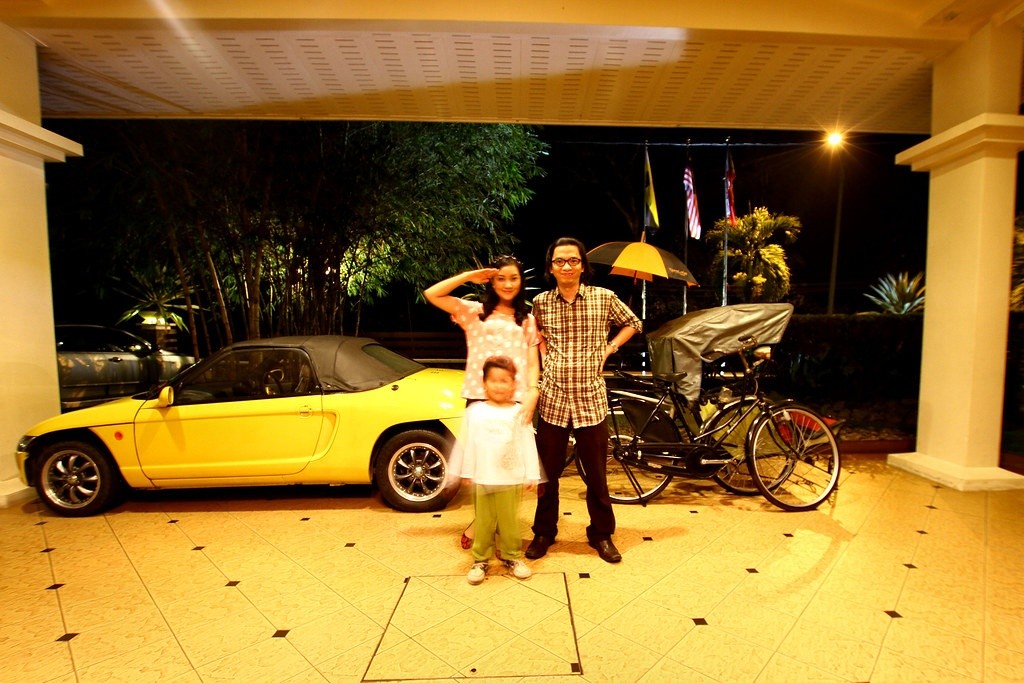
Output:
[602,369,744,380]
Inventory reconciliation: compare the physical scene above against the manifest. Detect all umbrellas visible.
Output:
[586,231,698,375]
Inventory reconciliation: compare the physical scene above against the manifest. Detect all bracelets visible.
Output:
[609,341,618,352]
[526,385,541,390]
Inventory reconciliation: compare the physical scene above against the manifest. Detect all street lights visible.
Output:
[827,132,845,315]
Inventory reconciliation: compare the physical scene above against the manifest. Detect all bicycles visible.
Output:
[563,335,841,512]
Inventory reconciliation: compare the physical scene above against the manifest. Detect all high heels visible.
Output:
[494,531,506,561]
[462,518,475,549]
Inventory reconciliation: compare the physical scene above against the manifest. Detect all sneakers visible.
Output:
[468,559,489,585]
[508,561,532,579]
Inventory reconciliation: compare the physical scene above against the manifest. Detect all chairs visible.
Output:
[238,364,319,396]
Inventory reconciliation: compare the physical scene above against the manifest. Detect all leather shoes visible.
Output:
[525,535,556,560]
[588,534,622,562]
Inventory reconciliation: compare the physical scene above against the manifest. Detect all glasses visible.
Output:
[552,257,582,267]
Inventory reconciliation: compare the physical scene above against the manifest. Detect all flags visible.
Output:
[643,150,659,227]
[684,151,701,239]
[726,149,735,226]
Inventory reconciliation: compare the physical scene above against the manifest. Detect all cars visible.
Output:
[14,335,467,512]
[55,325,203,409]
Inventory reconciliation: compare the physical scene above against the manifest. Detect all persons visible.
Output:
[423,256,541,548]
[525,237,643,563]
[445,357,541,584]
